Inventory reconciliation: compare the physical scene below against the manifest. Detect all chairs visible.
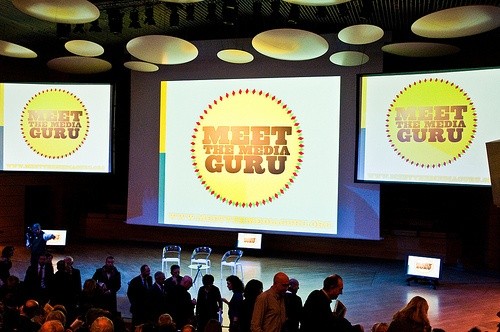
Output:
[161,245,181,275]
[220,250,243,279]
[191,247,212,277]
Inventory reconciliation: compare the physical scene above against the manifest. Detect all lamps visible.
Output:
[56,0,373,39]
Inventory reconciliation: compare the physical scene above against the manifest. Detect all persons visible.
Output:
[299,273,364,332]
[196,272,302,332]
[77,279,105,322]
[127,264,197,332]
[24,254,54,307]
[0,246,15,285]
[0,296,114,332]
[26,223,55,266]
[92,255,121,320]
[48,256,81,325]
[388,296,432,332]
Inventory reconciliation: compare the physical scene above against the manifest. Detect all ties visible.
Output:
[161,286,163,293]
[144,278,147,289]
[39,267,43,281]
